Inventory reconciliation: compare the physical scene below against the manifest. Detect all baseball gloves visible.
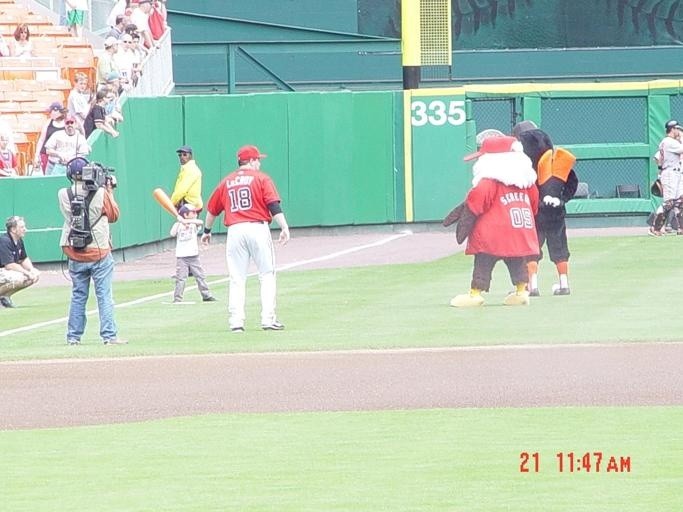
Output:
[653,179,664,197]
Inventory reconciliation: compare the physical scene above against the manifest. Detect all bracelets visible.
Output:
[204,227,211,236]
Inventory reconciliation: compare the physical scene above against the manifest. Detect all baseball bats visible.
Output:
[153,188,190,229]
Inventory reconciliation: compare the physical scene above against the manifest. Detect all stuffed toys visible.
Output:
[442,127,540,308]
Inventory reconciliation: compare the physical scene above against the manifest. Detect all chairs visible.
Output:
[0,1,109,181]
[571,181,589,201]
[615,183,641,200]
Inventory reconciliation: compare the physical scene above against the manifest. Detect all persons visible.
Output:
[31,0,167,176]
[0,133,20,177]
[6,23,35,59]
[509,119,579,296]
[199,144,292,334]
[167,145,204,278]
[168,202,218,304]
[56,156,129,346]
[0,215,42,308]
[645,119,682,238]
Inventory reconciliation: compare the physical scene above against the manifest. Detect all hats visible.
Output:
[235,142,269,161]
[178,203,203,216]
[176,144,193,155]
[665,117,683,130]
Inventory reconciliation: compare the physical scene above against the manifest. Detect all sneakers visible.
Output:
[105,335,130,346]
[260,320,286,331]
[203,297,219,303]
[648,227,683,237]
[0,295,16,310]
